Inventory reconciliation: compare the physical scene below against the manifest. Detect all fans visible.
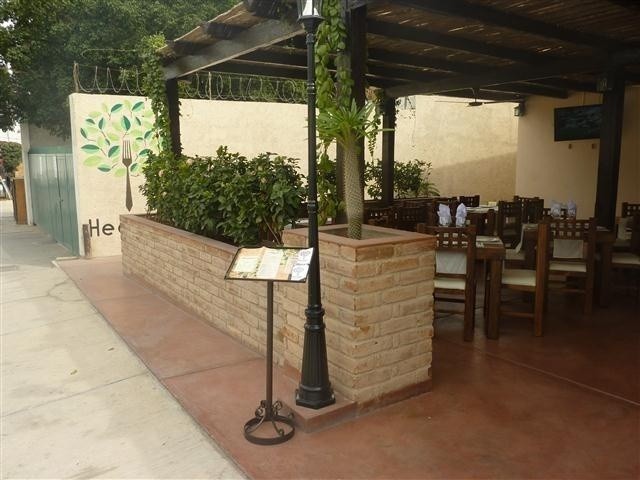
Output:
[434,87,526,108]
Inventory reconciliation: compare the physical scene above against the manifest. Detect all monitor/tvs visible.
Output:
[554,104,603,141]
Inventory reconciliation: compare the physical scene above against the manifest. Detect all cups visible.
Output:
[551,207,561,219]
[438,215,449,226]
[567,208,575,218]
[456,216,466,228]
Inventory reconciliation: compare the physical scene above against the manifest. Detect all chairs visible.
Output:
[288,193,639,343]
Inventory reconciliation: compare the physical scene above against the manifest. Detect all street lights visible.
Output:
[294,1,340,408]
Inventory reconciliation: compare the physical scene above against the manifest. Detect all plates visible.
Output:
[466,207,475,212]
[480,204,492,208]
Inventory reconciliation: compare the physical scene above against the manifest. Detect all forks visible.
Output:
[122,139,133,212]
